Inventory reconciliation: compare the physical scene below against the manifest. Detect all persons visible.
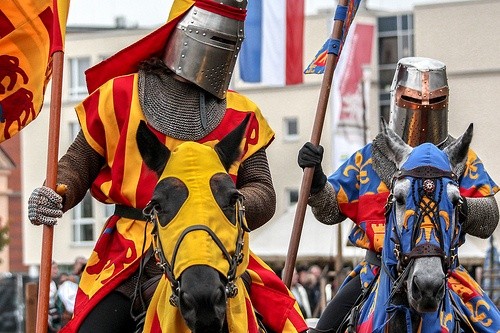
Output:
[281,265,351,319]
[299,56,500,333]
[48,256,87,333]
[28,1,308,333]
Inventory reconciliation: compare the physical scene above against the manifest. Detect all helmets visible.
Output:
[388,57,451,149]
[161,0,249,100]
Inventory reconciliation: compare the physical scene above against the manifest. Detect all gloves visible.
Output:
[298,141,328,192]
[28,184,69,227]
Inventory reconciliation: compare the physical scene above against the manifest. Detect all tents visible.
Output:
[250,201,485,264]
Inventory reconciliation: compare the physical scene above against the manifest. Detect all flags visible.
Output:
[0,0,70,143]
[239,0,305,85]
[331,15,376,171]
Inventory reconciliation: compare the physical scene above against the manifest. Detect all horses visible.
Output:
[339,115,474,333]
[139,141,264,331]
